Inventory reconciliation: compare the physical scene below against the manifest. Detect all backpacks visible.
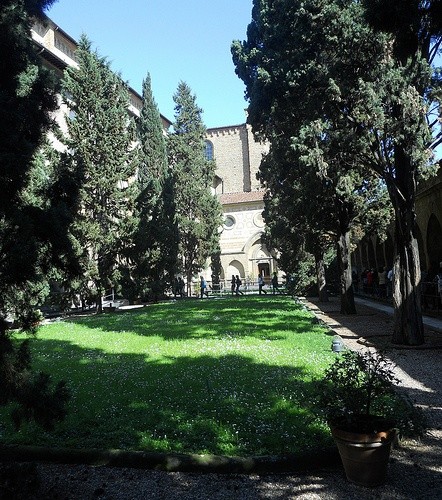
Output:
[436,275,442,293]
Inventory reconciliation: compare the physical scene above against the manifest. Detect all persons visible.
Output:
[231,275,243,296]
[258,273,267,295]
[173,277,185,297]
[272,272,281,295]
[200,276,207,298]
[350,266,393,300]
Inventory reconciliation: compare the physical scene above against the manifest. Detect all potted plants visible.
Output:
[311,338,429,488]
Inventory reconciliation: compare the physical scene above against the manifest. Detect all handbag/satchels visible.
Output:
[202,281,206,288]
[262,281,266,286]
